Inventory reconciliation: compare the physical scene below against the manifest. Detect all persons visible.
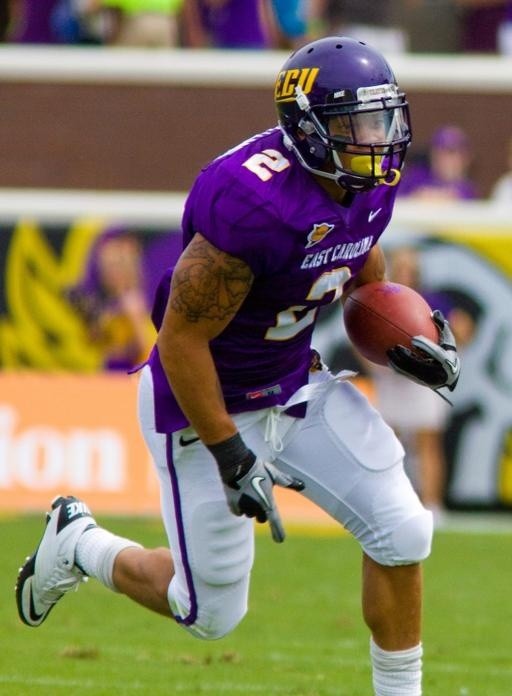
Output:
[392,123,481,322]
[14,35,462,696]
[61,222,160,371]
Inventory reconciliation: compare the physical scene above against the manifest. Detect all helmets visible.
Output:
[274,36,413,194]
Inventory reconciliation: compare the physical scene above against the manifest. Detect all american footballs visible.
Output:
[344,281,439,364]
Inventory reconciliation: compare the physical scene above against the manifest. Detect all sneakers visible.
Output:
[16,496,98,626]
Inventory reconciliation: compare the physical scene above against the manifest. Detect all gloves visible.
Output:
[205,432,305,543]
[385,309,461,391]
